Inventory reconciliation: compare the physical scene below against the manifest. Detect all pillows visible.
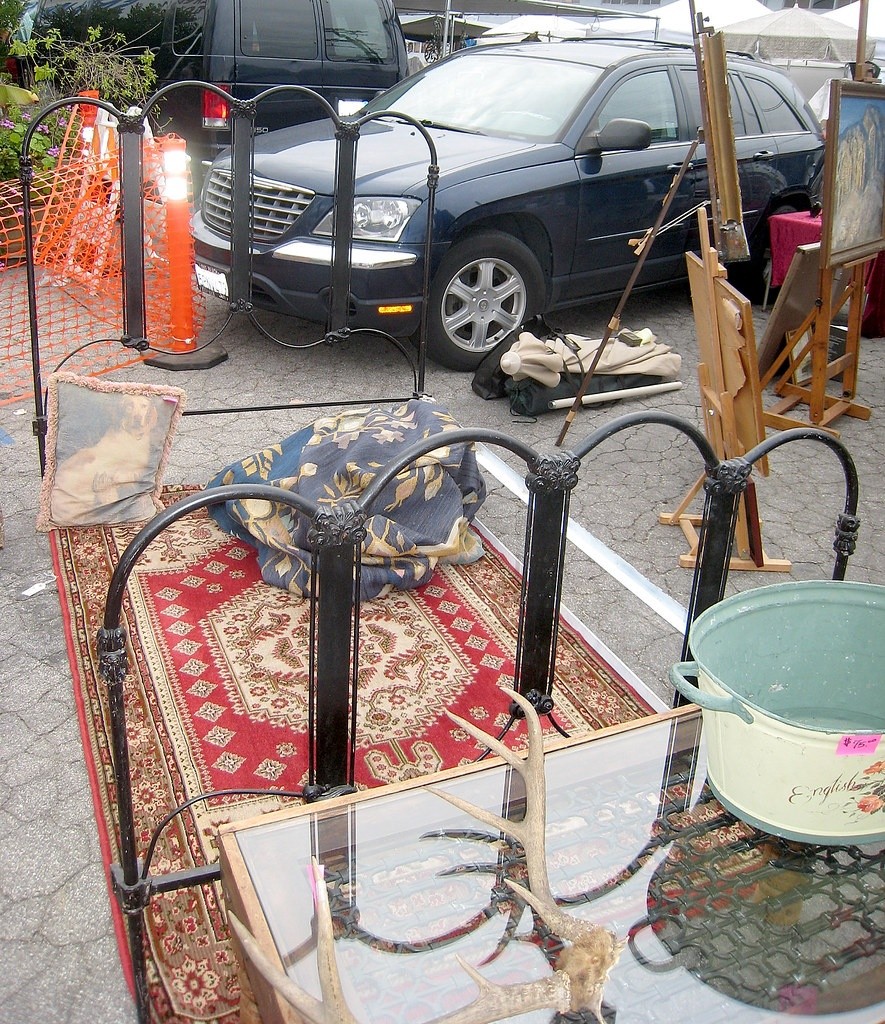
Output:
[35,372,188,533]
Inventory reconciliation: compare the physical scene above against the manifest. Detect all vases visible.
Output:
[0,205,59,259]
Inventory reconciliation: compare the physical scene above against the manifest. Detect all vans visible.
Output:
[14,2,409,206]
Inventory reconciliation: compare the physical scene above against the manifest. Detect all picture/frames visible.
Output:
[785,325,814,387]
[815,75,885,272]
[756,242,854,377]
[701,27,743,226]
[684,251,769,476]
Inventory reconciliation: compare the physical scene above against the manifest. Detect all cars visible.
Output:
[195,41,826,372]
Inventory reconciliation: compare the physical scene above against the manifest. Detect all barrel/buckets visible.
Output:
[670,580,885,847]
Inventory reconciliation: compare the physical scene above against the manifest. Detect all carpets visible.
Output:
[49,484,671,1024]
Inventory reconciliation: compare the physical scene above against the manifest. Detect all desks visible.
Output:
[762,211,885,332]
[217,703,885,1024]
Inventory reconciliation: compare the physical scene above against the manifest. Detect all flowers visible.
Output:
[0,106,73,202]
